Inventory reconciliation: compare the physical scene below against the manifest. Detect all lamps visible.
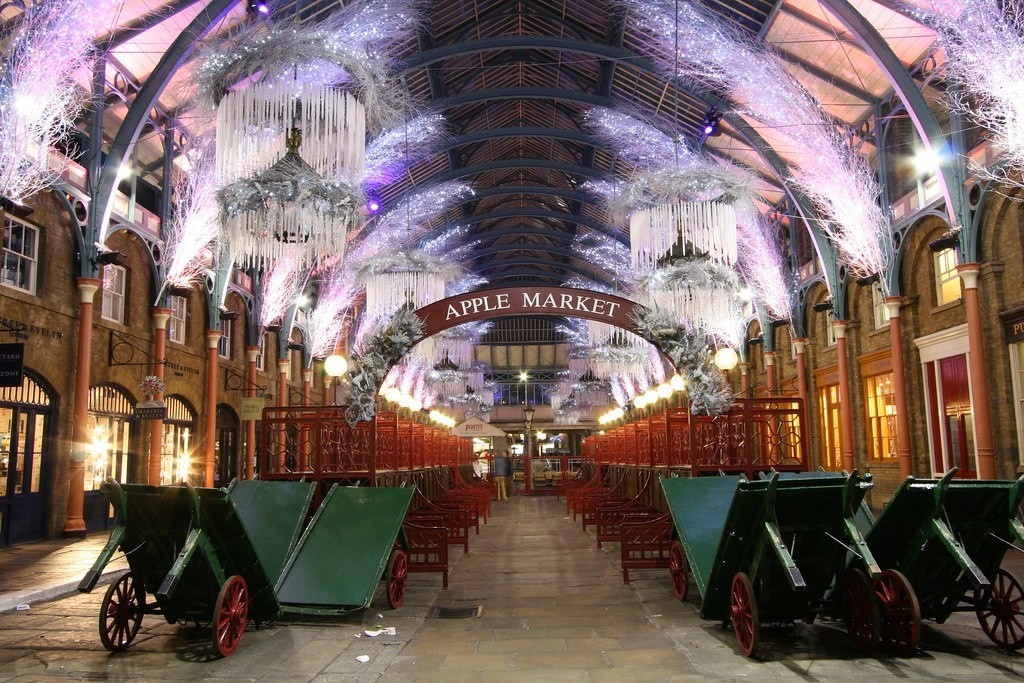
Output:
[287,343,304,350]
[928,238,956,253]
[748,337,762,345]
[95,250,120,265]
[523,404,535,432]
[812,301,832,312]
[702,111,722,136]
[219,311,241,321]
[769,318,788,327]
[854,274,879,286]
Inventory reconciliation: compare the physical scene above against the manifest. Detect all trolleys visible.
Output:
[77,476,321,657]
[819,466,1024,659]
[273,480,417,617]
[655,468,884,659]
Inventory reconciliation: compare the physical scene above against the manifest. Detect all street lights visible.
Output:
[518,371,528,404]
[522,405,535,456]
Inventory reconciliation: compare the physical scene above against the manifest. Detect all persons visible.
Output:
[473,449,513,500]
